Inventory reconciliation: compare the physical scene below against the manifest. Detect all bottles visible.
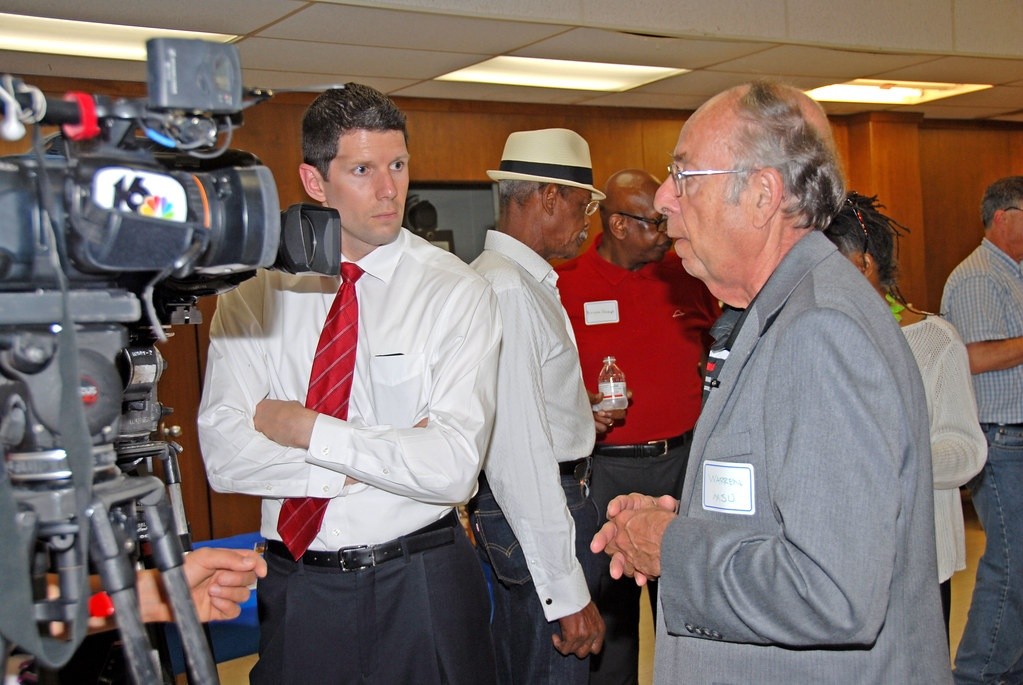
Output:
[598,356,628,420]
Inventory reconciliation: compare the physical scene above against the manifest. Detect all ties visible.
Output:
[701,346,730,414]
[276,262,365,562]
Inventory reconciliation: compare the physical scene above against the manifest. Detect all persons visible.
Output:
[822,192,990,655]
[546,168,716,685]
[940,175,1023,685]
[588,80,954,685]
[467,127,608,685]
[0,548,269,676]
[197,82,505,685]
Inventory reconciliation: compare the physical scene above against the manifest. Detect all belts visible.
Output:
[979,423,1023,428]
[263,506,459,574]
[590,429,694,459]
[558,457,593,480]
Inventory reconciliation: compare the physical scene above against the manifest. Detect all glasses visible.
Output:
[667,164,785,201]
[616,212,669,233]
[538,182,599,216]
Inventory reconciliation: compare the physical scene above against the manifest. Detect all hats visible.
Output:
[486,128,606,200]
